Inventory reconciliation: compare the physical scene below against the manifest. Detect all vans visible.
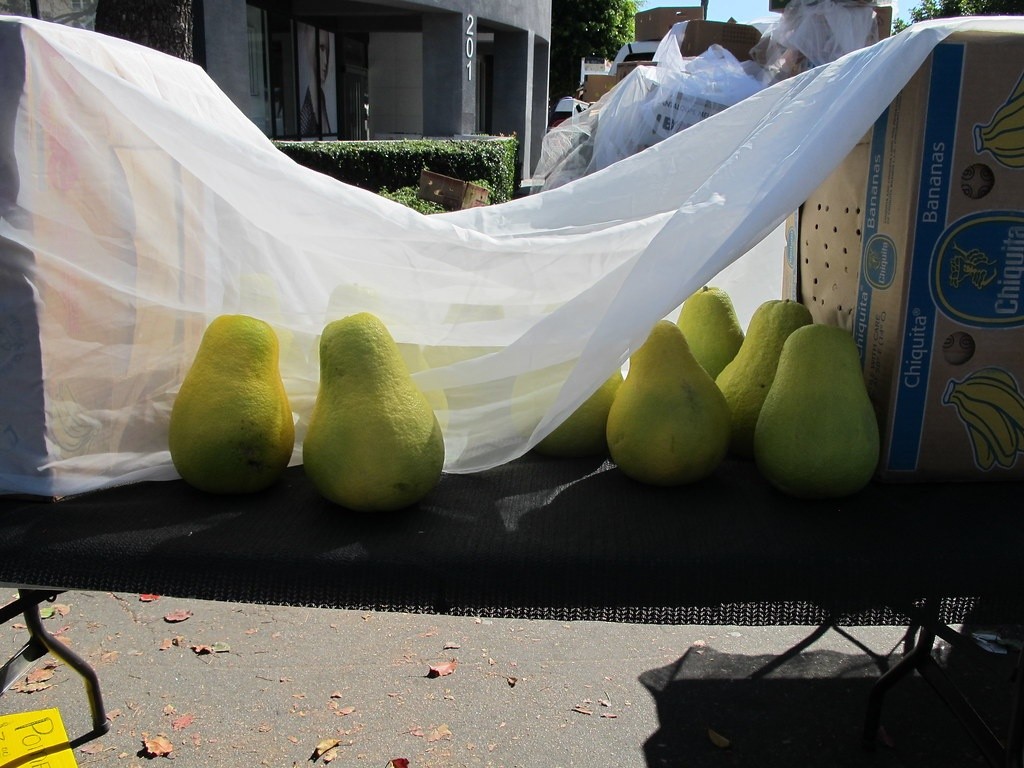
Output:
[609,40,661,75]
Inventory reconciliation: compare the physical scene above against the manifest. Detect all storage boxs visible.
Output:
[582,0,1024,474]
[0,17,224,502]
[418,170,490,212]
